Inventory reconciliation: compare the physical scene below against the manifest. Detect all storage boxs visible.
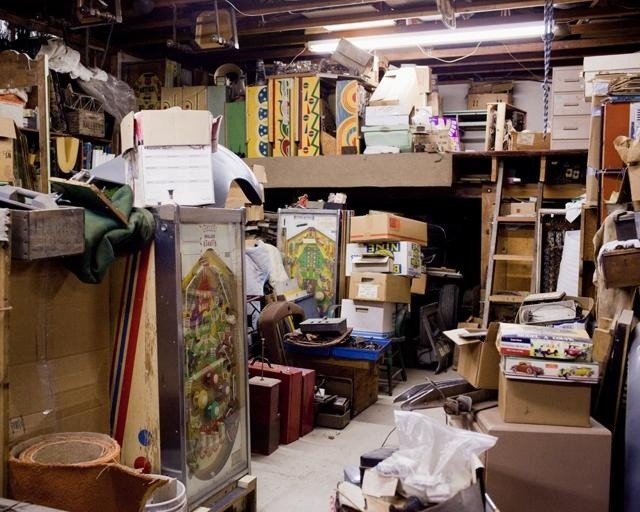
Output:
[340,299,397,340]
[498,370,592,428]
[350,214,428,247]
[1,240,113,503]
[349,272,412,304]
[344,240,422,280]
[0,117,16,183]
[362,63,549,153]
[329,446,491,512]
[442,322,500,390]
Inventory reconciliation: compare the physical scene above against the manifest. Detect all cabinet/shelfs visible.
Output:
[550,66,592,148]
[451,404,613,511]
